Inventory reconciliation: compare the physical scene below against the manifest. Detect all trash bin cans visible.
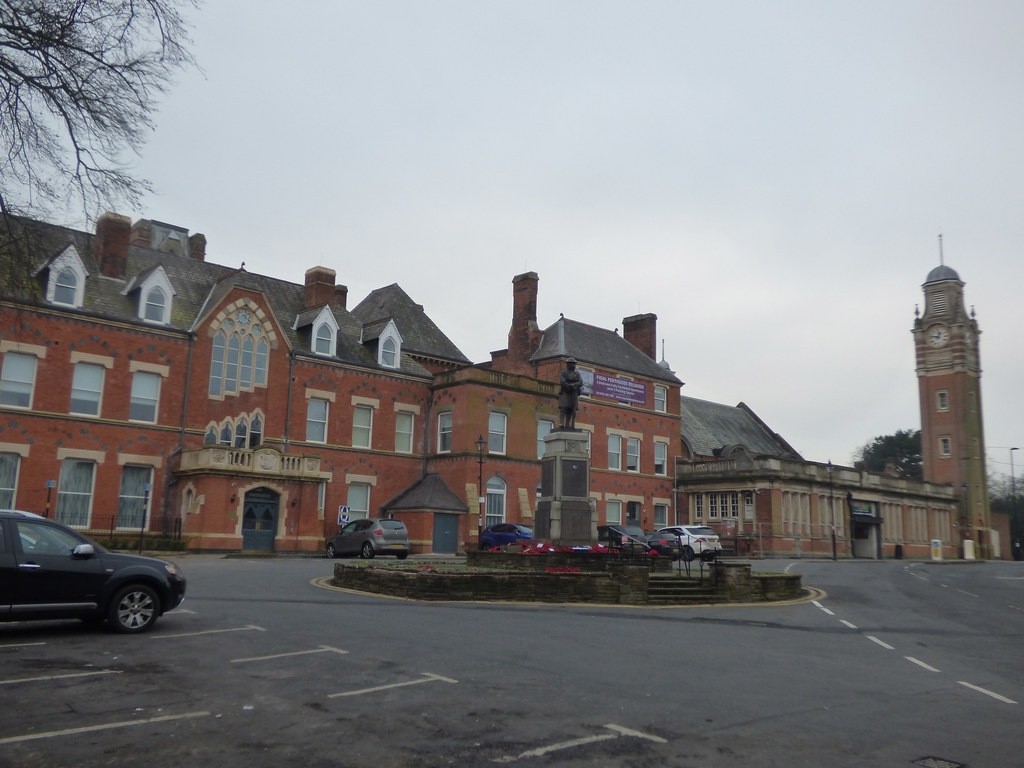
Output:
[895,544,903,559]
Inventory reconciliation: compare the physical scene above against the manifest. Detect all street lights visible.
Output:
[825,460,838,561]
[475,434,488,535]
[1010,447,1022,561]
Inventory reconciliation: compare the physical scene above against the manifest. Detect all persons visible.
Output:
[556,357,584,427]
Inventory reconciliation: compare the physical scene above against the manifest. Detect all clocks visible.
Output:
[924,322,949,349]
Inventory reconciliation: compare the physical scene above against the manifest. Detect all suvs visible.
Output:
[324,518,411,560]
[596,524,649,556]
[0,509,187,635]
[649,525,723,562]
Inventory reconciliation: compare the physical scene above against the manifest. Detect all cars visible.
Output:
[644,533,684,561]
[478,523,534,552]
